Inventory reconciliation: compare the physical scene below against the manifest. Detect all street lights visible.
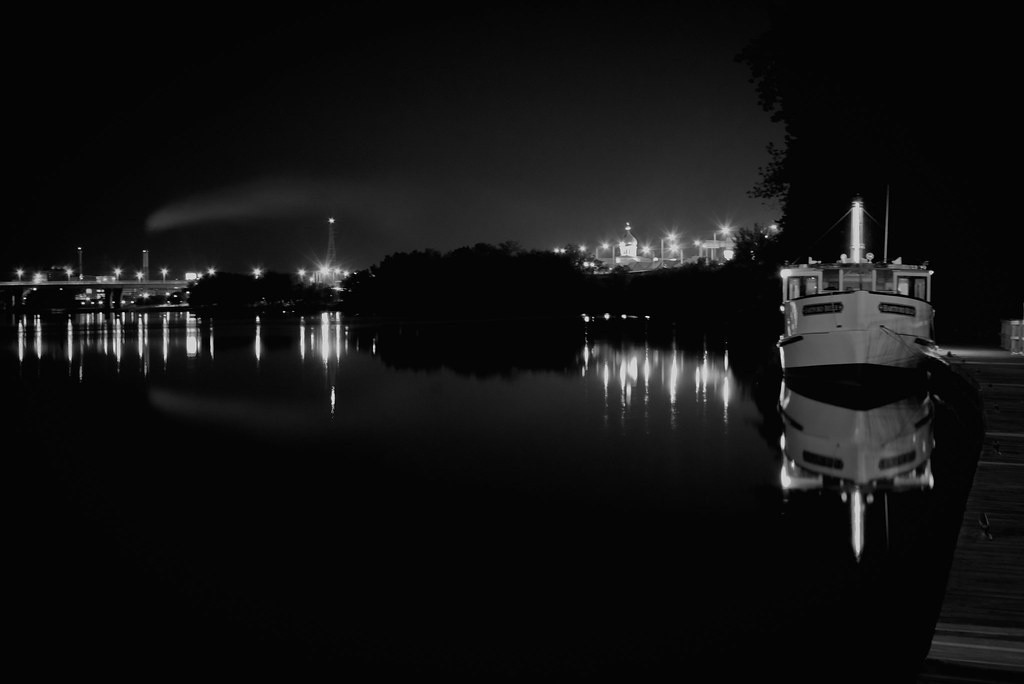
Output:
[17,266,168,284]
[554,227,734,272]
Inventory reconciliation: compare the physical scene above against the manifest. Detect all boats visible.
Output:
[773,365,934,564]
[777,183,937,374]
